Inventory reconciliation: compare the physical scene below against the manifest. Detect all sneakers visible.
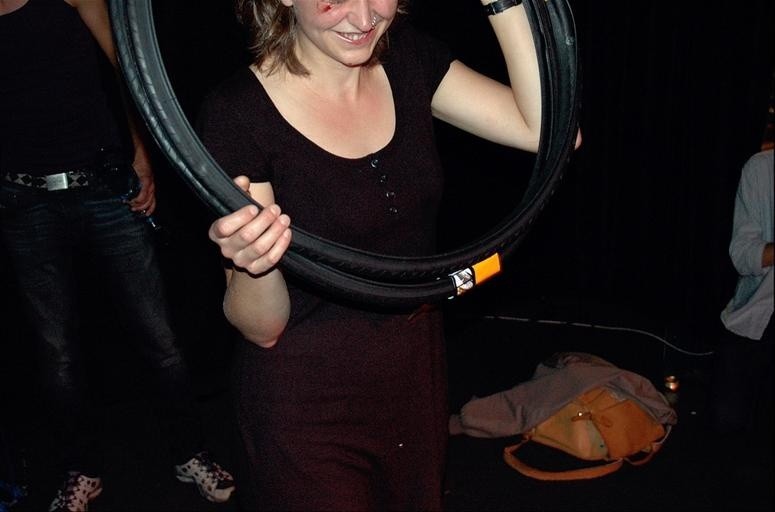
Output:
[176,452,236,503]
[47,474,102,511]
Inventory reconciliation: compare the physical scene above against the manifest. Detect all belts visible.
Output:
[5,169,90,190]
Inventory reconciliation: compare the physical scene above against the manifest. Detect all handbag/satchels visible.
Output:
[505,386,671,479]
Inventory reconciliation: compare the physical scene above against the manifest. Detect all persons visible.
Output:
[206,0,584,511]
[0,0,239,512]
[721,104,775,340]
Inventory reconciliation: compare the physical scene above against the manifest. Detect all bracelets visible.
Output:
[482,0,523,16]
